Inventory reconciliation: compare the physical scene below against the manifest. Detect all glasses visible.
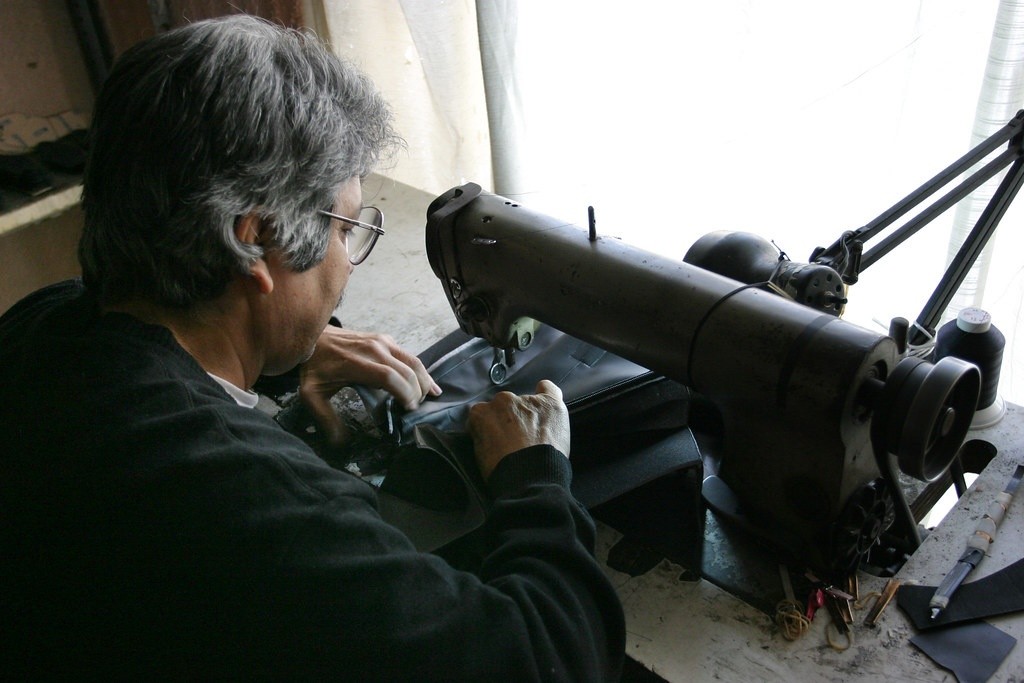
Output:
[318,206,385,265]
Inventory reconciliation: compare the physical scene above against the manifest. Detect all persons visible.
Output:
[0,11,628,683]
